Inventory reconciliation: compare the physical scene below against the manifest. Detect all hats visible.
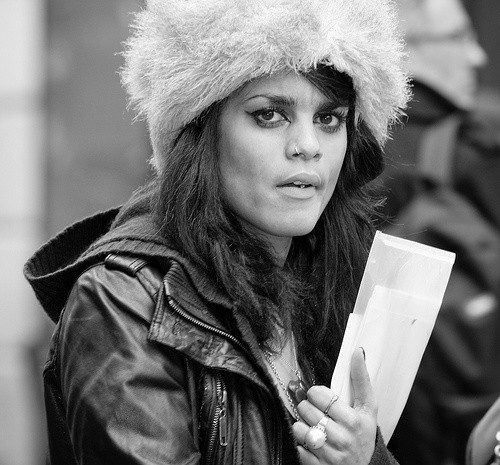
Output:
[114,0,415,174]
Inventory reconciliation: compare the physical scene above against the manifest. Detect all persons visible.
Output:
[22,1,399,465]
[362,1,500,465]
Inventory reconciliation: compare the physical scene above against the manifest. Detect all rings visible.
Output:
[303,426,327,450]
[315,416,329,432]
[323,395,339,414]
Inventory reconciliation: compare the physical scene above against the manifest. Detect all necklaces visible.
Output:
[265,329,309,404]
